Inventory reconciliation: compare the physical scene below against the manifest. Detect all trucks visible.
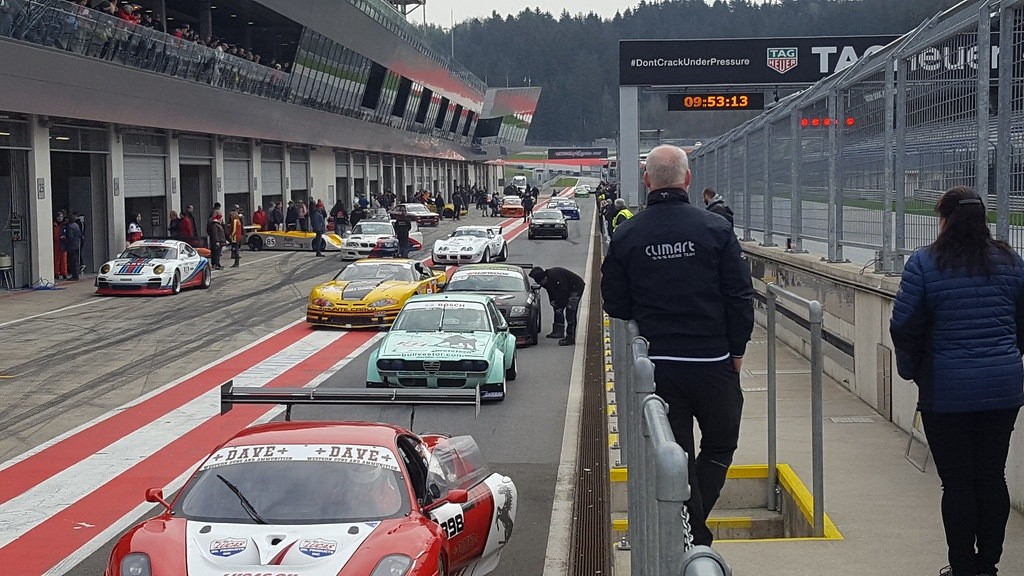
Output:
[512,175,527,190]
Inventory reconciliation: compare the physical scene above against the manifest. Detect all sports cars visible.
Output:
[306,257,447,330]
[574,185,591,197]
[366,293,519,403]
[243,225,342,251]
[106,381,517,576]
[500,196,525,217]
[431,226,508,265]
[97,238,212,296]
[440,262,541,349]
[341,219,422,260]
[555,201,580,220]
[424,204,479,217]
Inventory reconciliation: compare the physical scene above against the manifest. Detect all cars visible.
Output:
[526,210,569,240]
[387,203,439,227]
[547,196,568,209]
[363,208,391,220]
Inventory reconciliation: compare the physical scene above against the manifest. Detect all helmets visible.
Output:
[460,308,482,329]
[346,453,383,483]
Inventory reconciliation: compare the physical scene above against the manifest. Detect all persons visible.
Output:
[350,203,367,229]
[354,189,396,209]
[127,212,145,243]
[330,198,348,238]
[528,266,586,346]
[549,189,560,197]
[889,186,1024,576]
[435,191,445,221]
[399,184,434,205]
[59,0,289,86]
[207,202,245,269]
[594,181,617,237]
[503,183,522,199]
[461,309,481,328]
[253,199,309,232]
[169,205,199,249]
[519,182,539,223]
[54,209,86,282]
[309,197,327,258]
[703,186,734,230]
[394,214,411,259]
[601,144,756,552]
[452,185,500,221]
[487,230,492,238]
[612,199,634,232]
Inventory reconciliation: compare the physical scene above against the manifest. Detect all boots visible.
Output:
[547,323,576,346]
[230,257,240,268]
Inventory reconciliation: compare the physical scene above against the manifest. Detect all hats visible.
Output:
[287,200,294,204]
[234,204,239,209]
[361,194,365,197]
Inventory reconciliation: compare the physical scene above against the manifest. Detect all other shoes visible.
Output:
[239,249,243,251]
[940,569,953,576]
[212,264,224,271]
[453,215,459,221]
[491,214,499,217]
[482,215,488,217]
[316,253,326,257]
[67,276,79,281]
[63,275,68,279]
[54,276,59,280]
